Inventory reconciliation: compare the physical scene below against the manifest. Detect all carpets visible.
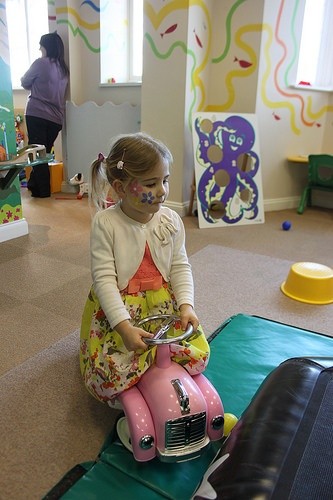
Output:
[0,242,333,500]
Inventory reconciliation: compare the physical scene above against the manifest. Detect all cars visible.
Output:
[115,313,227,463]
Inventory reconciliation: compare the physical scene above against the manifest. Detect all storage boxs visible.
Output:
[25,162,64,194]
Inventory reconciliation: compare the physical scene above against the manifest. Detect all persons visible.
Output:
[77,132,210,403]
[19,33,70,198]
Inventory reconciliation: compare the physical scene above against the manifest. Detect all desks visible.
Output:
[287,156,312,208]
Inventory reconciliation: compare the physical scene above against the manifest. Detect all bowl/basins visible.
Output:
[281,262,333,304]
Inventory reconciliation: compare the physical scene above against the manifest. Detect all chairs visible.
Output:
[296,153,333,214]
